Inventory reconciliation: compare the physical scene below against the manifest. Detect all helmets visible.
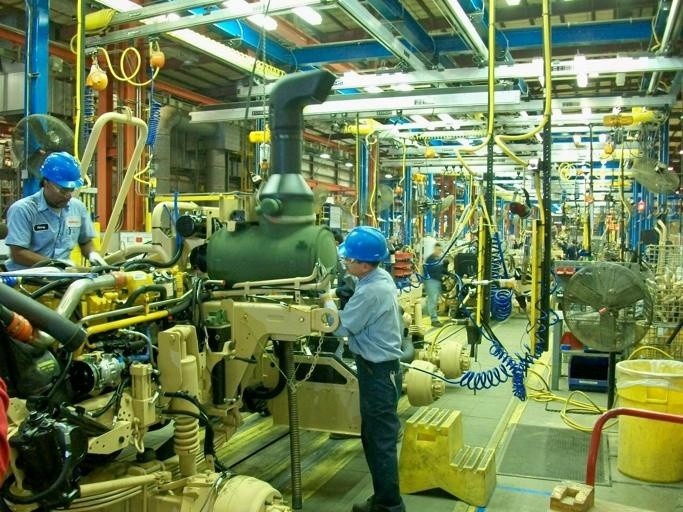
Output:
[337,226,389,262]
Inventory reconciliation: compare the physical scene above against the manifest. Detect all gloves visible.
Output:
[89,251,108,267]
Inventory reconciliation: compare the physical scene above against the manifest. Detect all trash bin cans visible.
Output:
[615,358,683,483]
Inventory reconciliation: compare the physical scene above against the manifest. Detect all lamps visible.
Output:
[312,184,327,209]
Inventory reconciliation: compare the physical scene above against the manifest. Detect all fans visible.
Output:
[632,158,680,195]
[376,183,394,214]
[437,194,454,216]
[564,261,653,418]
[12,114,74,179]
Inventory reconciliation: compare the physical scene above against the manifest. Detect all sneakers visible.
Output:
[353,495,405,512]
[431,321,443,327]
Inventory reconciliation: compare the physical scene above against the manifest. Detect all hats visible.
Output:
[39,152,85,188]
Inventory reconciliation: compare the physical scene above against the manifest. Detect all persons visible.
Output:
[334,234,343,250]
[0,152,109,273]
[566,240,580,260]
[424,244,443,328]
[332,226,405,512]
